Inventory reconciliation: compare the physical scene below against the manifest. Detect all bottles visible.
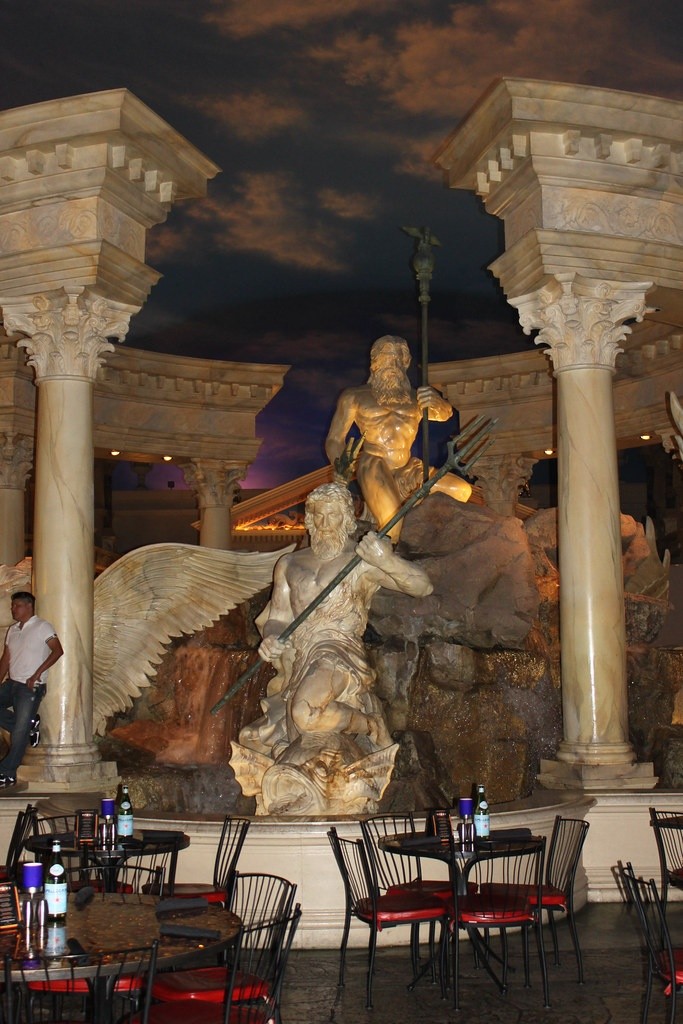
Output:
[41,920,66,969]
[44,839,67,920]
[473,784,490,840]
[118,785,133,840]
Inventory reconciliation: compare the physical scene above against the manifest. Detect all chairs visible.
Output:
[130,901,305,1024]
[448,832,552,1013]
[143,812,252,908]
[326,825,446,1011]
[647,807,683,948]
[0,803,38,880]
[151,869,298,1001]
[478,814,591,987]
[61,861,164,896]
[3,934,162,1024]
[33,811,135,894]
[356,806,478,978]
[620,863,683,1024]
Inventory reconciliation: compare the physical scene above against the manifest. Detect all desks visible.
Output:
[0,890,243,1024]
[24,828,192,897]
[376,830,546,992]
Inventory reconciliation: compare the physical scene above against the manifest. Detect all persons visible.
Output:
[324,335,472,543]
[0,591,64,790]
[238,481,434,755]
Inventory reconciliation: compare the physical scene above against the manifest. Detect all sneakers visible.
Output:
[29,714,40,747]
[0,774,16,790]
